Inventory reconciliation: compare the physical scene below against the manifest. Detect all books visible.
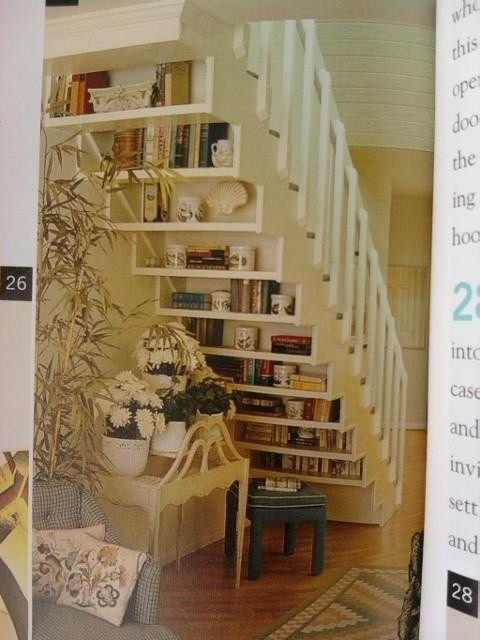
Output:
[172,300,210,310]
[271,345,311,356]
[52,59,113,117]
[153,61,191,106]
[204,355,361,478]
[139,122,236,172]
[172,291,212,303]
[271,334,311,346]
[185,241,230,270]
[228,280,279,314]
[179,317,224,346]
[145,183,171,224]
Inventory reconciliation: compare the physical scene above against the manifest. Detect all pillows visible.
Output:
[33,523,149,628]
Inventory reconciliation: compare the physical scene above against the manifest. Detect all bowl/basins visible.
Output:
[211,154,233,167]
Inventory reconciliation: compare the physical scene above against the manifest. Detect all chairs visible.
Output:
[33,478,180,640]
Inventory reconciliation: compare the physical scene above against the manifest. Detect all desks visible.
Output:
[84,418,251,595]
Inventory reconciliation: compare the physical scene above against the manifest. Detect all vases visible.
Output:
[98,436,152,478]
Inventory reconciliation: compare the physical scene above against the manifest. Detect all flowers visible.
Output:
[89,319,237,436]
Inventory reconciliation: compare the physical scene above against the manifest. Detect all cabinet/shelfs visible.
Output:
[40,56,376,488]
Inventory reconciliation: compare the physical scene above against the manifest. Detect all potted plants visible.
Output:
[161,378,235,442]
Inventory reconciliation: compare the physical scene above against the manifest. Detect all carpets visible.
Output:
[255,563,418,640]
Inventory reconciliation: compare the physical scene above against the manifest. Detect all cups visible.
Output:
[212,139,233,154]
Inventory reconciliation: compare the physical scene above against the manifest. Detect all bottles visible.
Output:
[234,326,259,351]
[270,294,293,315]
[176,196,205,223]
[273,364,297,387]
[229,246,256,271]
[164,244,187,268]
[287,400,305,419]
[210,290,232,313]
